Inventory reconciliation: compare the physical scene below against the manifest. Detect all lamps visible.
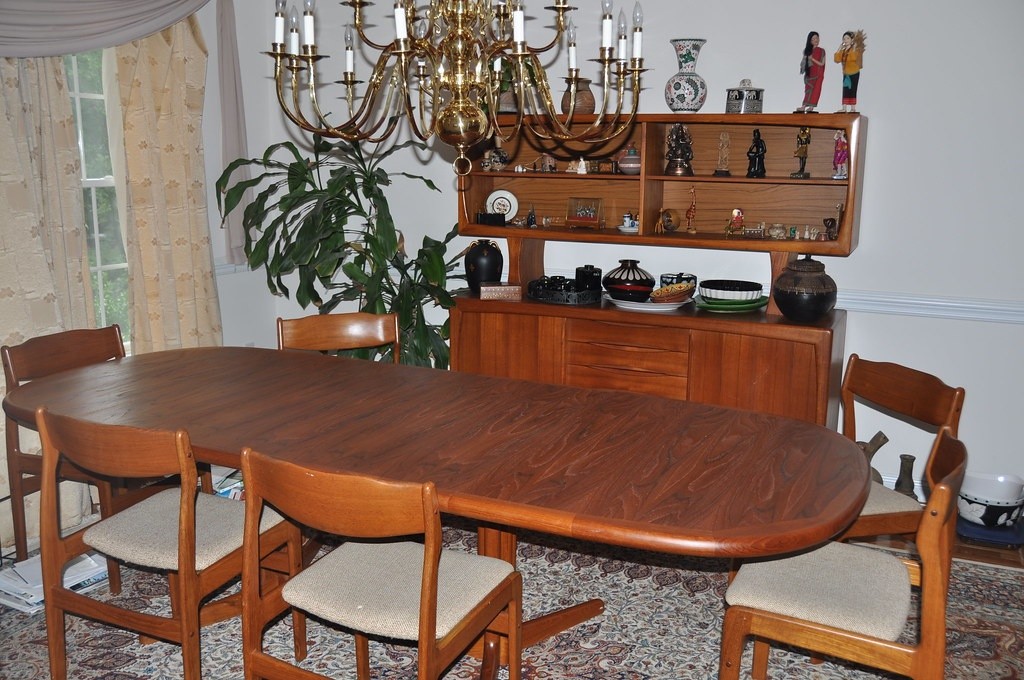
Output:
[260,0,654,175]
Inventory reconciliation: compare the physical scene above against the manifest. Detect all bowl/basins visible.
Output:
[699,280,762,300]
[661,274,697,297]
[957,473,1024,527]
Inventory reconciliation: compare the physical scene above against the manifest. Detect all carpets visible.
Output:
[0,524,1024,680]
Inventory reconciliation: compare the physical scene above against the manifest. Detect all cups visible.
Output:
[624,214,630,227]
[819,233,828,241]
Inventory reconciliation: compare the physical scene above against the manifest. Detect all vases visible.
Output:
[562,78,595,114]
[895,455,918,501]
[601,259,655,303]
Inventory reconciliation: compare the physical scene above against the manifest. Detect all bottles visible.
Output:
[664,38,707,114]
[768,223,786,237]
[602,259,655,302]
[465,240,503,293]
[480,136,508,172]
[803,229,810,238]
[618,149,640,174]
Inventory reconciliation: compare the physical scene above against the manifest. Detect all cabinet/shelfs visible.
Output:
[451,113,869,432]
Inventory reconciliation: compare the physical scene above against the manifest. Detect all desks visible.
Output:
[2,346,872,666]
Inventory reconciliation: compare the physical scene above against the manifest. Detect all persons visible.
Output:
[834,30,866,112]
[833,129,847,176]
[747,129,767,173]
[796,31,825,112]
[793,126,811,173]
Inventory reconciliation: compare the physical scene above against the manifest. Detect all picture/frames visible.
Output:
[665,39,707,113]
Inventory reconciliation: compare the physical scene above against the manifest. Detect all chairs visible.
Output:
[240,446,524,680]
[276,313,400,364]
[0,324,213,595]
[719,424,968,680]
[730,352,966,583]
[36,406,307,680]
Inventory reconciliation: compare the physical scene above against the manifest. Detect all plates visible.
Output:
[485,190,518,221]
[695,297,770,313]
[603,293,693,310]
[618,225,638,232]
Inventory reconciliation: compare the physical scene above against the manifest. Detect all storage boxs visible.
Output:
[479,282,522,302]
